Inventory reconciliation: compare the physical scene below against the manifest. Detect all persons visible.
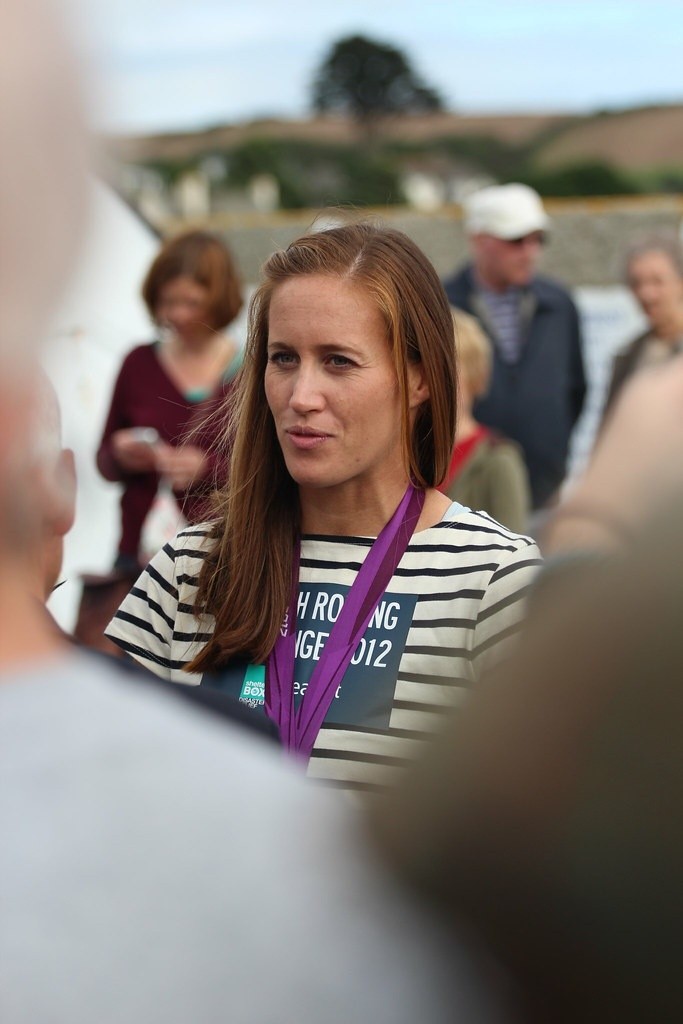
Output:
[432,304,529,539]
[96,232,249,588]
[363,483,682,1024]
[440,184,591,515]
[101,222,545,811]
[0,334,500,1024]
[585,237,683,478]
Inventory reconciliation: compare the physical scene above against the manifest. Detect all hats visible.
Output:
[460,182,551,240]
